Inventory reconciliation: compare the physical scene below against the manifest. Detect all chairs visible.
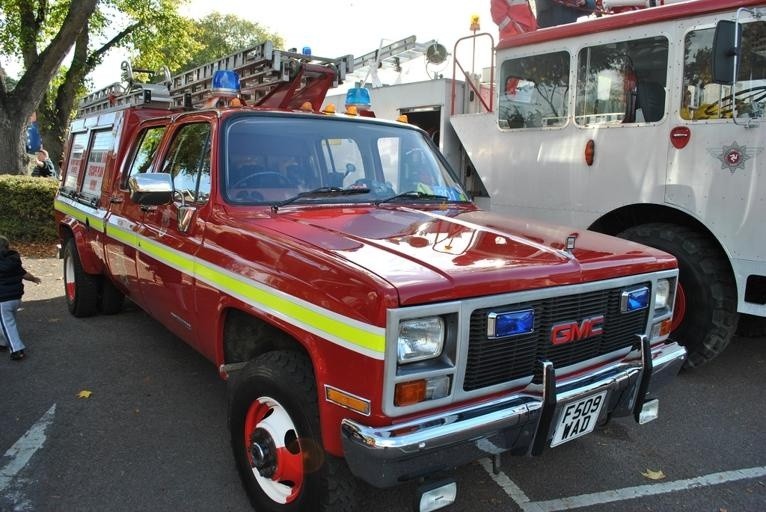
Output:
[636,81,665,122]
[238,164,321,186]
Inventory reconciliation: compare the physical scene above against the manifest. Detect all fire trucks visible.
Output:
[48,30,689,512]
[320,2,766,372]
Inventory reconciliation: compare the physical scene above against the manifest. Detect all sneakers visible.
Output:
[10,350,24,359]
[0,345,7,351]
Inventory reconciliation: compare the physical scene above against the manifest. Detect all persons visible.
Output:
[491,0,541,42]
[31,149,57,178]
[0,235,42,360]
[534,0,597,28]
